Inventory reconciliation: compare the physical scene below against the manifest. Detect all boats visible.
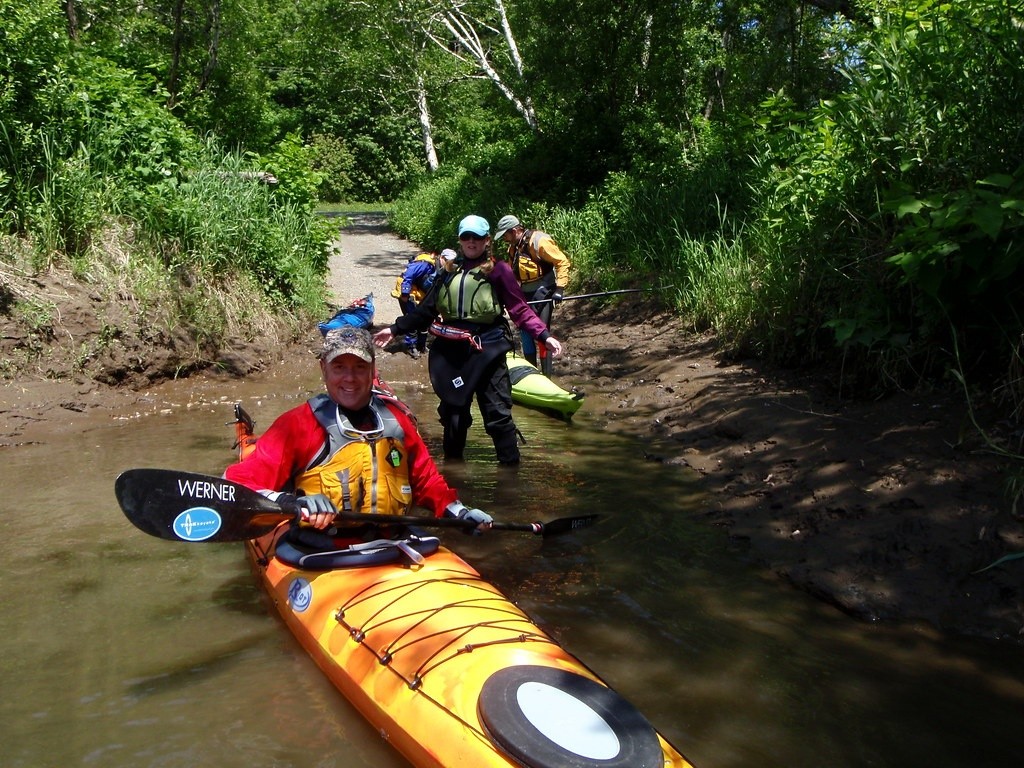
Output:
[489,349,587,425]
[232,404,700,768]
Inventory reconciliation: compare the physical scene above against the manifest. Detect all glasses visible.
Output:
[459,232,488,241]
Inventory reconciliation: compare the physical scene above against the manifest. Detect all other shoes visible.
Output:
[416,345,430,353]
[406,347,421,359]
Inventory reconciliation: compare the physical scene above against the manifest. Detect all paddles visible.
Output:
[115,467,608,543]
[525,284,676,305]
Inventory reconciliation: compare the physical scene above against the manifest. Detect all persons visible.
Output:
[371,216,562,470]
[494,216,570,379]
[391,248,457,359]
[221,327,494,553]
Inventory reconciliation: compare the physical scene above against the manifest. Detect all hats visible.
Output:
[494,215,519,241]
[313,327,375,363]
[440,249,458,260]
[459,215,491,236]
